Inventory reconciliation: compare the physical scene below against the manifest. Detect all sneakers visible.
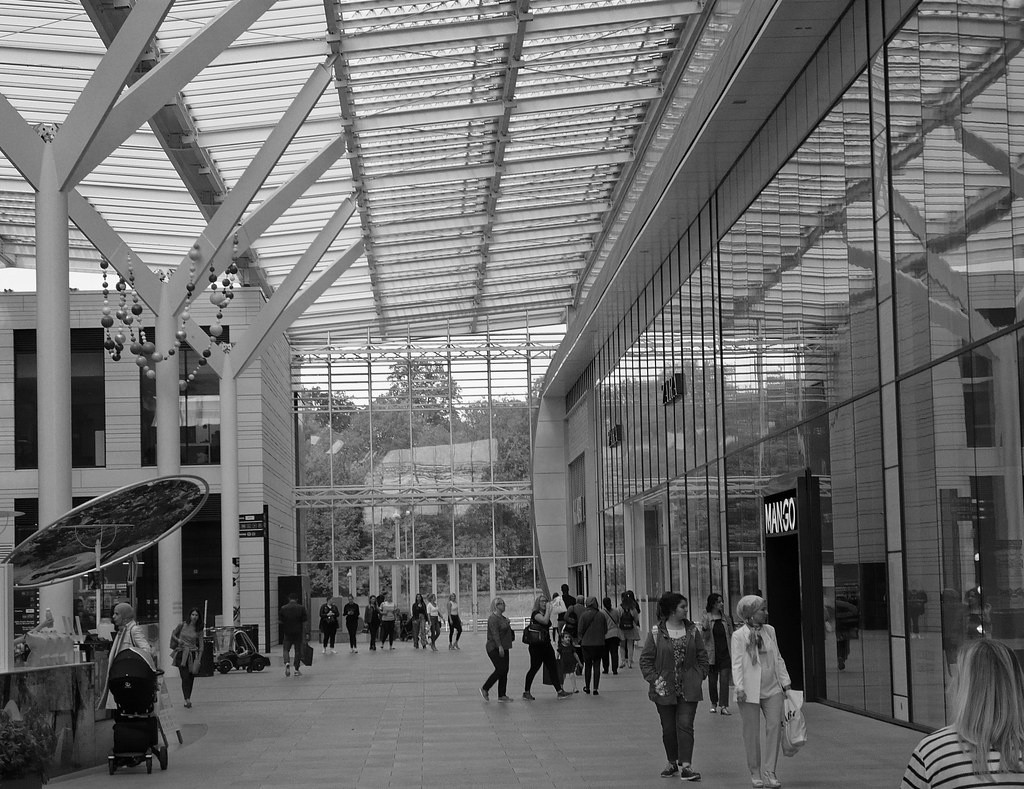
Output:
[660,762,700,780]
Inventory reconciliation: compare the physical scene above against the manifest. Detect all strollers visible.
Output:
[105,645,169,774]
[398,611,416,642]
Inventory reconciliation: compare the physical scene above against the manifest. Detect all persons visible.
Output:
[319,596,340,654]
[97,602,150,720]
[478,598,514,704]
[277,593,309,677]
[940,588,992,674]
[426,593,445,651]
[835,593,859,669]
[909,582,927,639]
[412,594,428,649]
[522,582,641,701]
[701,593,734,716]
[342,594,360,653]
[730,596,791,788]
[900,638,1024,789]
[172,608,204,709]
[447,592,462,650]
[14,618,54,645]
[363,590,402,651]
[639,592,710,781]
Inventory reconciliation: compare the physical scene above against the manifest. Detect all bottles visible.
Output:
[14,643,28,653]
[32,630,86,666]
[328,610,332,618]
[98,618,115,641]
[46,608,53,628]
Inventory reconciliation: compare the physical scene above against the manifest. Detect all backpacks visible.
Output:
[620,606,634,629]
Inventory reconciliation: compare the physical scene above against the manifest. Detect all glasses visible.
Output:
[498,602,505,605]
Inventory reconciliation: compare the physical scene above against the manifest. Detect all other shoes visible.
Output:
[184,702,192,708]
[350,649,358,653]
[763,771,781,788]
[709,703,717,713]
[429,641,437,651]
[285,663,303,676]
[721,706,732,715]
[478,659,634,703]
[751,774,763,787]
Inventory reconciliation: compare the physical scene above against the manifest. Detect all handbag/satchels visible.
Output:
[522,611,544,643]
[511,629,515,641]
[170,622,185,649]
[781,690,807,757]
[300,639,313,666]
[543,658,564,685]
[562,606,578,638]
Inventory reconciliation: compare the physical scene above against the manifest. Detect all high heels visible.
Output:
[448,643,461,650]
